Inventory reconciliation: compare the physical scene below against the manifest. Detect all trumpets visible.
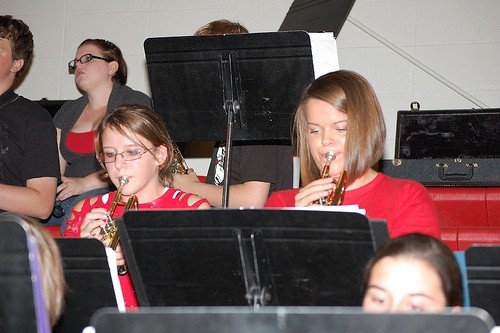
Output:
[99,176,139,251]
[310,151,349,206]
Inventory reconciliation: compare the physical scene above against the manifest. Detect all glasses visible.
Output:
[96,147,153,163]
[67,54,110,67]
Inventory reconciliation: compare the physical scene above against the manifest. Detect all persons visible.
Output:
[263,69,441,242]
[0,13,62,218]
[354,231,465,314]
[51,38,153,236]
[62,104,210,312]
[3,208,65,325]
[163,18,293,208]
